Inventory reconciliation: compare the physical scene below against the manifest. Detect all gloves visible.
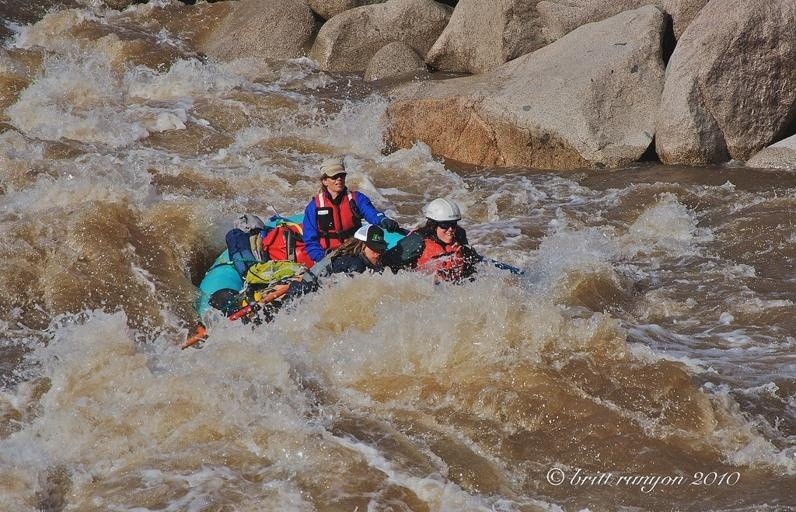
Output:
[382,219,399,233]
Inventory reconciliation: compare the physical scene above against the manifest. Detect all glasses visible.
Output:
[436,222,457,229]
[325,174,346,180]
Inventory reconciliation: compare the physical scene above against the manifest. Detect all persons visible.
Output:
[279,224,389,308]
[302,157,399,263]
[381,197,484,286]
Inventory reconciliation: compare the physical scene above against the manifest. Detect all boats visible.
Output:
[194,213,525,338]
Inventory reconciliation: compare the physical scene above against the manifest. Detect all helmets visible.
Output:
[426,198,461,221]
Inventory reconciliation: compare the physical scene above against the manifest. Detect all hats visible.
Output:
[320,160,348,177]
[354,224,387,251]
